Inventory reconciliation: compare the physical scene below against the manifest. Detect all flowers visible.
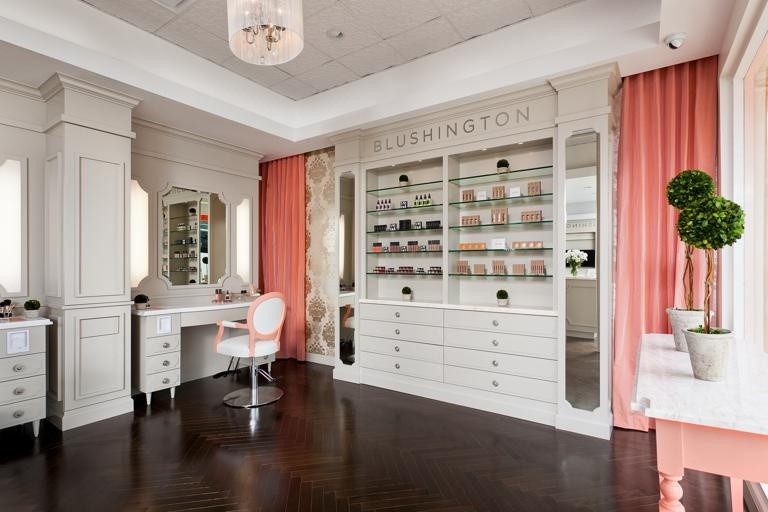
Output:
[563,248,590,267]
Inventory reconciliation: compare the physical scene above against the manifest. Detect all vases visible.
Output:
[570,266,579,278]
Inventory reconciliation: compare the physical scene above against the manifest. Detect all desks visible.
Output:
[339,289,355,309]
[565,275,600,342]
[130,297,278,407]
[629,331,768,511]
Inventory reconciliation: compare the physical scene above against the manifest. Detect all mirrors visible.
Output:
[337,169,356,366]
[563,126,604,414]
[156,181,231,291]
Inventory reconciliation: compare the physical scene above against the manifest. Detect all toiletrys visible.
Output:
[212,284,256,304]
[168,217,198,272]
[372,194,441,275]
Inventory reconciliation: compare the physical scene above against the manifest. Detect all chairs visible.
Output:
[341,301,356,364]
[212,289,289,410]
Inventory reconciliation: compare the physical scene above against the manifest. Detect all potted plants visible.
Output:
[401,286,413,301]
[188,208,196,216]
[664,169,719,355]
[496,158,509,174]
[677,192,747,384]
[135,293,147,309]
[23,299,41,318]
[188,279,196,284]
[398,174,408,187]
[496,288,510,307]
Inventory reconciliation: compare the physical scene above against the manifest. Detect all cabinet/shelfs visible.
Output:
[0,315,55,440]
[162,192,225,284]
[365,180,444,276]
[444,307,560,428]
[358,302,445,405]
[447,164,555,279]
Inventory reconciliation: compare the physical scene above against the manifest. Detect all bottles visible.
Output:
[369,192,441,275]
[173,220,196,272]
[214,283,260,303]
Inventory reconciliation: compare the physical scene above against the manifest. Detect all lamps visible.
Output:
[224,1,306,69]
